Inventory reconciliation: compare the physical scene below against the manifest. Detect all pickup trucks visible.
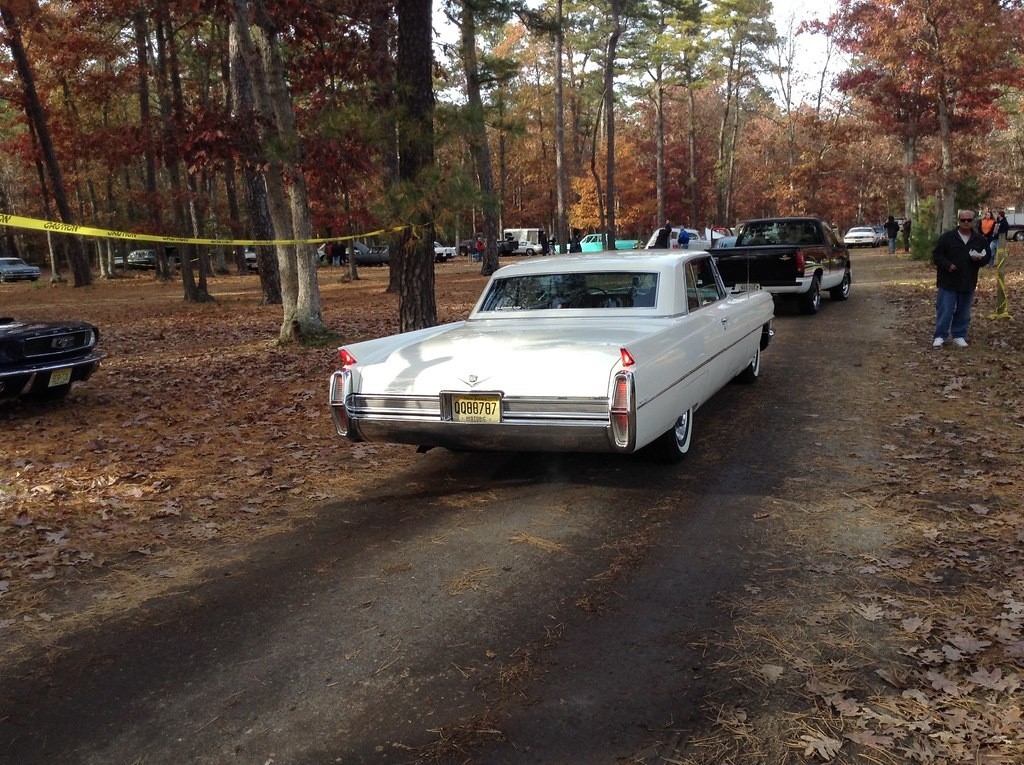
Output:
[705,216,854,314]
[554,234,644,254]
[460,233,519,256]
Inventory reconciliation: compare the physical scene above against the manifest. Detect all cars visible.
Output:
[644,227,734,248]
[328,248,775,464]
[0,257,42,282]
[0,316,109,414]
[354,243,389,267]
[316,242,326,261]
[512,241,542,255]
[434,242,457,262]
[114,245,182,270]
[843,217,910,247]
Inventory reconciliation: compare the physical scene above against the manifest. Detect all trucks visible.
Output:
[994,213,1024,242]
[503,228,549,244]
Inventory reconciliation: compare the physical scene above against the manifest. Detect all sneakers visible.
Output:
[933,337,944,347]
[952,336,969,348]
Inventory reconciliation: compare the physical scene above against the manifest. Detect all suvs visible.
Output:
[232,246,259,274]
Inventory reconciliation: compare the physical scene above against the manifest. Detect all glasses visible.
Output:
[959,218,974,223]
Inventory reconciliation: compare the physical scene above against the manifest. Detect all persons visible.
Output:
[323,241,346,268]
[475,236,485,262]
[541,231,556,256]
[977,210,1009,268]
[931,210,990,349]
[901,216,911,254]
[883,215,900,254]
[648,223,689,250]
[569,228,583,254]
